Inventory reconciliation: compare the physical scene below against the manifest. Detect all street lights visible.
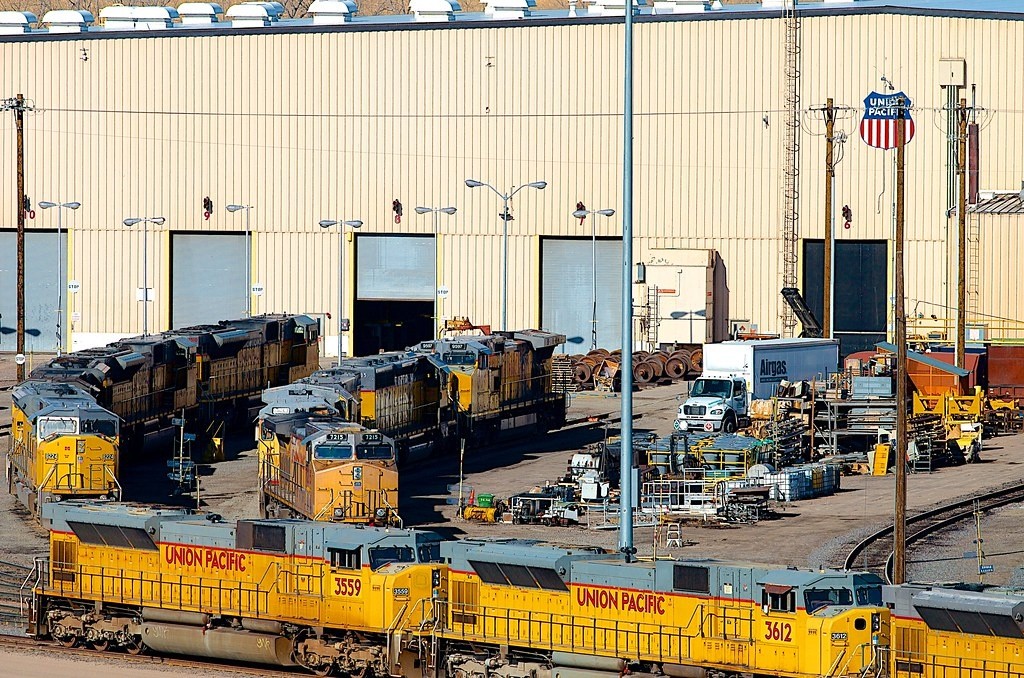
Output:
[572,210,616,350]
[225,204,254,318]
[38,202,83,358]
[318,220,363,367]
[415,206,458,341]
[123,217,167,335]
[465,180,547,331]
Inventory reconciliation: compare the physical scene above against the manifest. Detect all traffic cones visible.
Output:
[468,490,475,505]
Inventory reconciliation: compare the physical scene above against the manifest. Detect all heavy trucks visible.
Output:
[677,338,839,435]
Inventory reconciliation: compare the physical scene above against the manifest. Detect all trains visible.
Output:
[7,311,319,517]
[19,499,1024,678]
[258,330,568,528]
